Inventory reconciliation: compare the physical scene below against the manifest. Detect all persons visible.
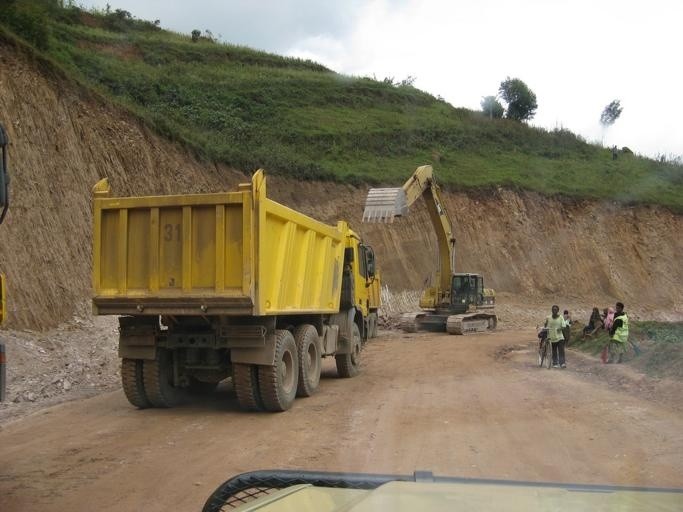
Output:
[579,306,615,342]
[543,304,567,369]
[611,144,618,160]
[605,302,629,364]
[562,309,574,342]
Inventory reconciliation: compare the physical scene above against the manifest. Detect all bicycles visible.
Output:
[536,324,553,369]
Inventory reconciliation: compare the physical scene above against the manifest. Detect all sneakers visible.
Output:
[551,363,565,369]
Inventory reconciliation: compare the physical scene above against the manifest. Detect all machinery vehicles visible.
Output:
[361,164,498,335]
